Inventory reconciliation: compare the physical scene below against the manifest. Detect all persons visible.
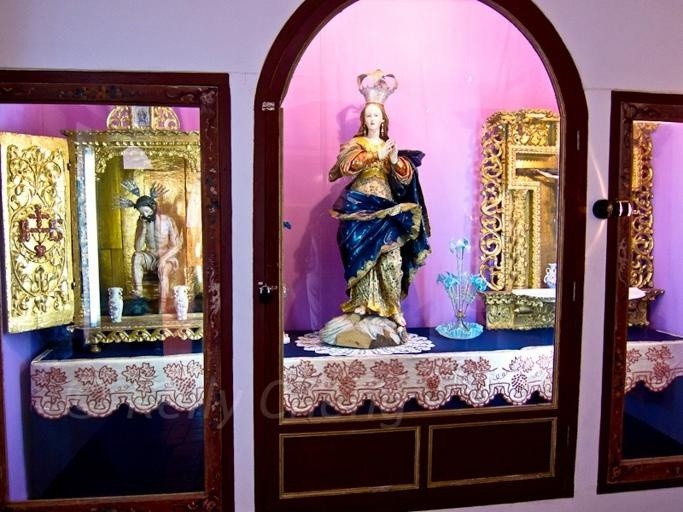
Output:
[128,196,181,314]
[329,70,433,326]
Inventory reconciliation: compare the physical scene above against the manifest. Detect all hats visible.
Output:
[356,68,399,107]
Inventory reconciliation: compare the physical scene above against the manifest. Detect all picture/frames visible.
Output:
[2,127,203,345]
[251,0,589,510]
[1,69,234,512]
[597,89,683,496]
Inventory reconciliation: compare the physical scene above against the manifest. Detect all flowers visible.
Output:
[433,237,489,339]
